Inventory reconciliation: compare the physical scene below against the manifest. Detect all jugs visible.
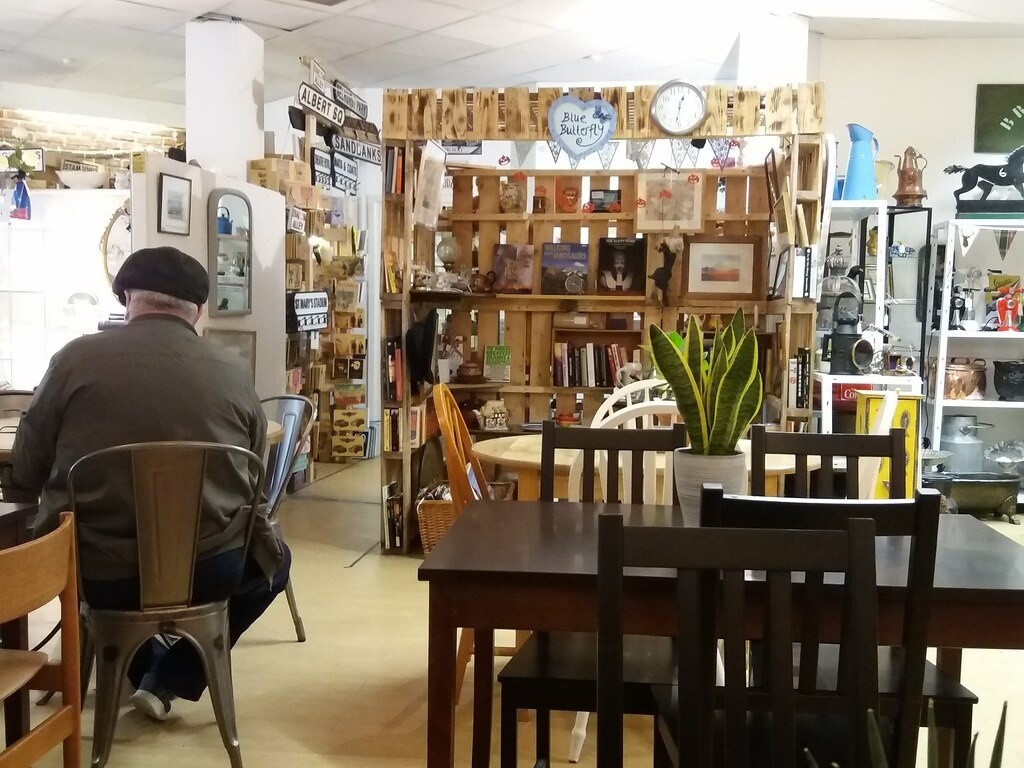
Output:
[893,146,928,208]
[841,123,879,201]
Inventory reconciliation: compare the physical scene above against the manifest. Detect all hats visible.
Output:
[112,246,210,307]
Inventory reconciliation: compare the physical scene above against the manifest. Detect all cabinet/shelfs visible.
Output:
[373,137,1024,558]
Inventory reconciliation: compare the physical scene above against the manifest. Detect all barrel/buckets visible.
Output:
[942,414,994,472]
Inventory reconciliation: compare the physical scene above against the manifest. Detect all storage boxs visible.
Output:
[334,408,369,430]
[332,434,366,456]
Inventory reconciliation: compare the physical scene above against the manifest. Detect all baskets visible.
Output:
[414,480,516,556]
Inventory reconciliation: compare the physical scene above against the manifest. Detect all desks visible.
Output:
[1,412,283,702]
[415,499,1024,765]
[470,432,826,505]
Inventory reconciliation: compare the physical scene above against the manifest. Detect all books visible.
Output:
[788,348,811,433]
[554,340,641,390]
[483,344,511,383]
[490,237,648,297]
[386,146,405,194]
[383,250,402,294]
[383,481,403,551]
[381,336,439,451]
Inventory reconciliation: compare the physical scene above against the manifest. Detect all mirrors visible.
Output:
[207,187,253,318]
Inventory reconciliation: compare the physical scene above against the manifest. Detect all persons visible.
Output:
[11,247,291,721]
[505,245,533,289]
[598,250,638,292]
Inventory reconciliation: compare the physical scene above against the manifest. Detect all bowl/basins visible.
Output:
[922,449,953,474]
[53,169,108,191]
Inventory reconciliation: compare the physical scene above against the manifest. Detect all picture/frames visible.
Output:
[157,172,193,236]
[631,171,707,231]
[680,232,765,300]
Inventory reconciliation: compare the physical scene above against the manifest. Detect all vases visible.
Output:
[12,168,31,220]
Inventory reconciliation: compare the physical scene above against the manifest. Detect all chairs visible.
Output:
[253,392,317,645]
[62,440,264,768]
[431,382,536,724]
[496,380,979,768]
[1,507,81,767]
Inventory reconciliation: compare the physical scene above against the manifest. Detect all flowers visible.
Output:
[6,125,35,177]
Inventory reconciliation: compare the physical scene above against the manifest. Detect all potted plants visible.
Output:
[648,307,765,528]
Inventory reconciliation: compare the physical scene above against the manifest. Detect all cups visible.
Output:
[533,195,550,212]
[502,182,523,213]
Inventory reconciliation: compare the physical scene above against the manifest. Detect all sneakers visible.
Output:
[130,672,171,720]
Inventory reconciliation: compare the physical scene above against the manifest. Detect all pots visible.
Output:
[992,360,1024,402]
[944,473,1023,524]
[930,357,987,401]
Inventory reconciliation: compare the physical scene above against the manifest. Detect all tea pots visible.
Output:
[456,359,484,377]
[472,398,511,431]
[216,206,234,234]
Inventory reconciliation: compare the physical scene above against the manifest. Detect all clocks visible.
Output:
[650,80,709,137]
